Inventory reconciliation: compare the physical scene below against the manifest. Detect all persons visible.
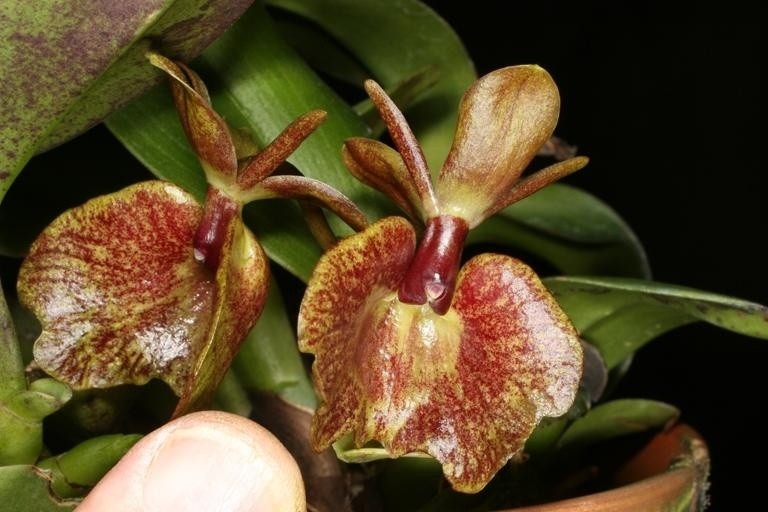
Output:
[68,408,309,511]
